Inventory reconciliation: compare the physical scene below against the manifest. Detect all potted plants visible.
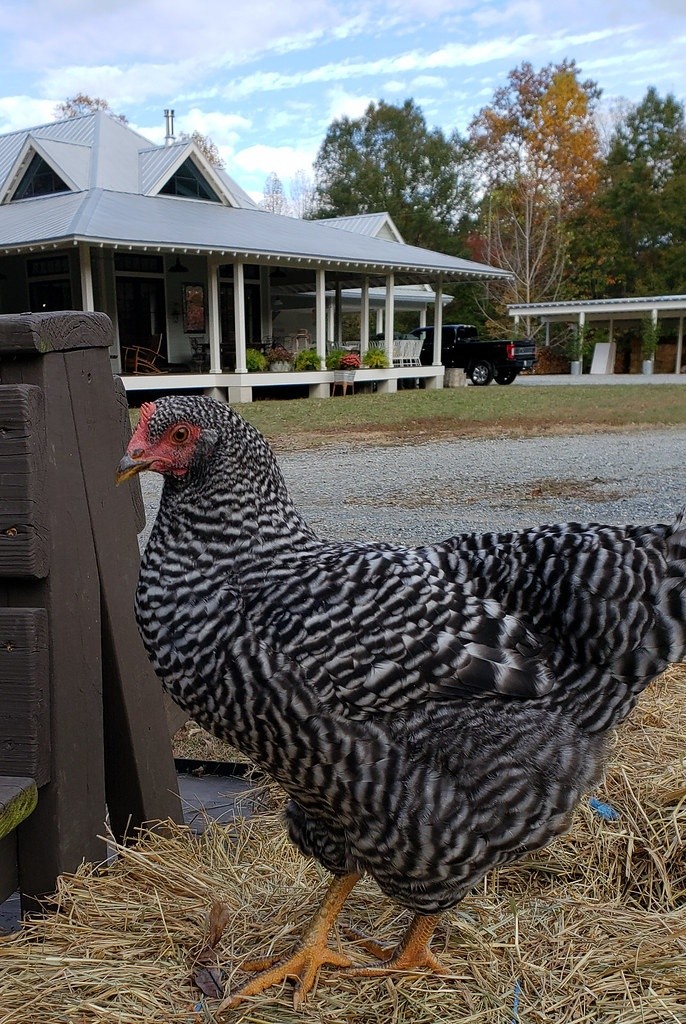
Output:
[570,320,594,375]
[265,346,293,373]
[246,349,264,373]
[640,310,662,374]
[364,348,388,368]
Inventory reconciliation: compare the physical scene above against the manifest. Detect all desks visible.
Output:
[198,343,270,372]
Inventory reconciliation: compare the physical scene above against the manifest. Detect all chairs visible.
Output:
[179,336,210,374]
[122,334,166,375]
[283,334,360,354]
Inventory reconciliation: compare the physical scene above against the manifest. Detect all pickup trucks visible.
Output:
[406,322,538,387]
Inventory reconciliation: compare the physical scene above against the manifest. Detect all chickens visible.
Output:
[113,394,686,1017]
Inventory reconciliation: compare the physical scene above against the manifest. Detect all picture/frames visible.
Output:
[181,281,207,334]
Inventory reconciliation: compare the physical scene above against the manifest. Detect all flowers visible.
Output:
[342,355,361,368]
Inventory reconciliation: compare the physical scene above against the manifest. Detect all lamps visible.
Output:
[168,254,189,274]
